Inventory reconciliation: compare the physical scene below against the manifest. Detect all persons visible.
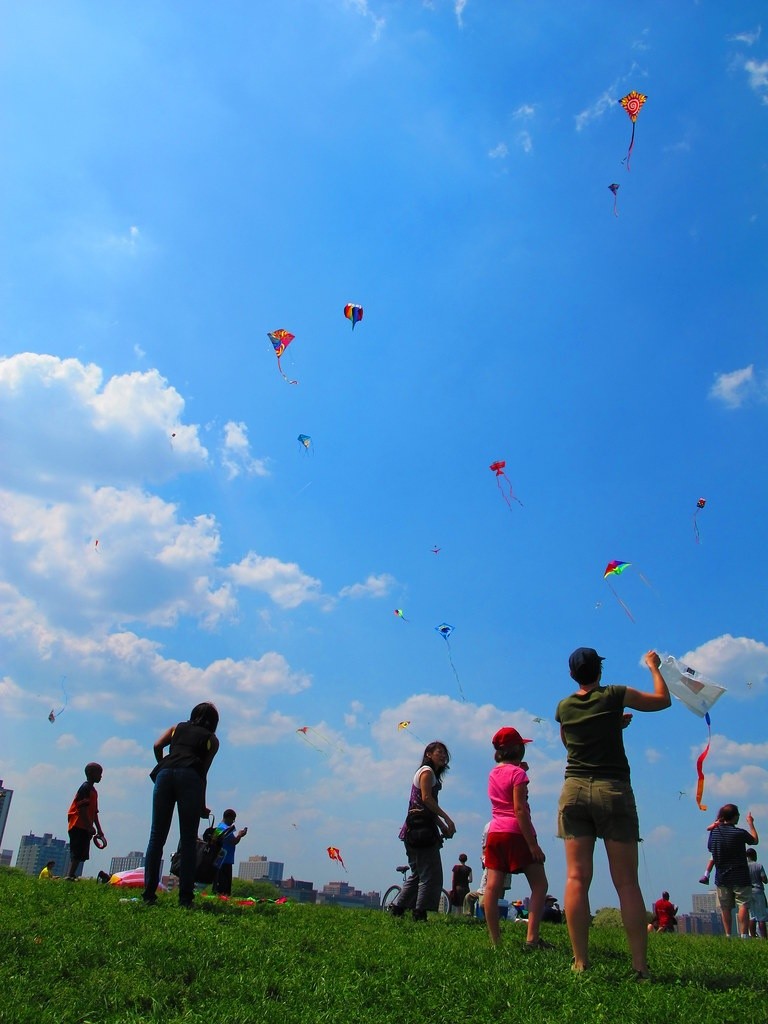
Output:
[38,861,60,879]
[483,727,558,953]
[746,847,768,939]
[541,895,562,924]
[212,808,247,896]
[463,820,512,916]
[698,813,724,886]
[555,647,673,980]
[388,742,457,922]
[707,803,759,938]
[654,891,677,933]
[141,702,220,910]
[66,762,103,880]
[449,853,472,914]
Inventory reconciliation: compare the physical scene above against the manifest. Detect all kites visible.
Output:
[48,676,68,723]
[267,329,298,385]
[603,560,664,623]
[618,90,649,172]
[656,653,728,812]
[691,498,707,545]
[489,459,524,511]
[393,609,407,621]
[434,622,467,703]
[426,543,444,560]
[327,846,347,872]
[343,303,364,331]
[608,181,622,217]
[296,727,346,760]
[297,434,314,457]
[398,720,426,746]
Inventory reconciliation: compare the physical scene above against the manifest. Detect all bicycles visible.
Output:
[381,866,452,914]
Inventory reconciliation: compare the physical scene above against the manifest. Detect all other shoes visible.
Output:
[699,876,709,885]
[634,965,657,987]
[413,909,428,921]
[523,939,556,955]
[390,904,405,916]
[569,955,590,972]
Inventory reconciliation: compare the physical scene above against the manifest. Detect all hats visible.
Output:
[570,648,606,682]
[546,895,558,902]
[492,727,533,749]
